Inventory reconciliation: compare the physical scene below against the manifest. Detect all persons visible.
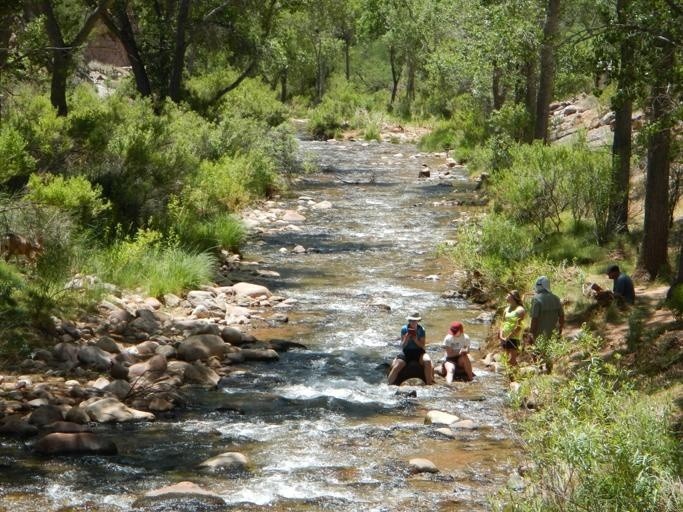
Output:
[590,265,634,305]
[440,321,473,384]
[386,310,434,385]
[526,276,564,345]
[497,289,526,365]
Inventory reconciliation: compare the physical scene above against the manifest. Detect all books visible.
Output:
[405,327,418,339]
[590,283,603,292]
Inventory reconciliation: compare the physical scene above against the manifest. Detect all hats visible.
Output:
[508,289,521,302]
[605,264,620,274]
[404,310,422,322]
[446,321,464,337]
